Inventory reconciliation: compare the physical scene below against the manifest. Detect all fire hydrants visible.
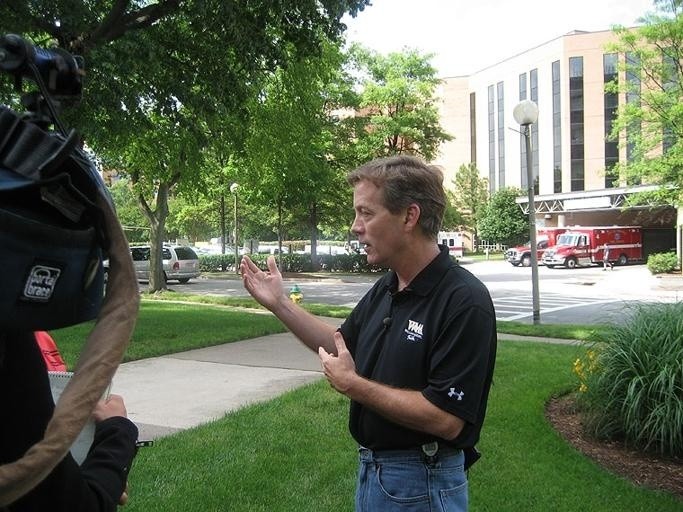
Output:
[289,284,303,307]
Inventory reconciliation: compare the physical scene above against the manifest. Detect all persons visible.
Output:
[1,333,138,512]
[603,243,613,271]
[241,156,498,512]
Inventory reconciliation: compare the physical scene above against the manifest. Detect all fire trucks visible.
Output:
[503,226,571,267]
[540,225,643,269]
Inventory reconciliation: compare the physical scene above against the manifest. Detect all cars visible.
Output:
[102,244,201,284]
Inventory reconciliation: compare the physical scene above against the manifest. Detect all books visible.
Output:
[48,371,113,466]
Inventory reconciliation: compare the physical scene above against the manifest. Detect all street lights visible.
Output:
[230,182,240,274]
[512,98,541,326]
